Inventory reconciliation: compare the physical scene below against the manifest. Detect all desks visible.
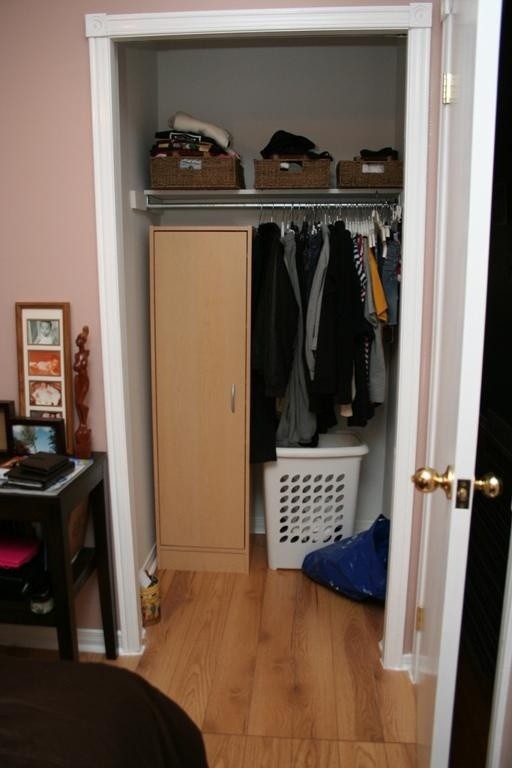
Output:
[0,451,119,661]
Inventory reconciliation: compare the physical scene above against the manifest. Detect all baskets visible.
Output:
[261,428,370,570]
[336,160,402,187]
[254,158,330,189]
[149,154,246,189]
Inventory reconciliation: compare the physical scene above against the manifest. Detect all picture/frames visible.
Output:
[6,415,66,457]
[14,302,76,456]
[0,399,15,460]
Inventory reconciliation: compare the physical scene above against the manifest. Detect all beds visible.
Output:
[0,656,209,767]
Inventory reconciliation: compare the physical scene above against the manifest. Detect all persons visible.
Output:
[28,355,60,377]
[33,319,58,346]
[33,381,55,405]
[73,332,90,433]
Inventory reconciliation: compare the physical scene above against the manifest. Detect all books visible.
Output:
[1,451,95,498]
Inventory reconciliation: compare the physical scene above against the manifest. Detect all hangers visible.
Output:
[255,198,402,259]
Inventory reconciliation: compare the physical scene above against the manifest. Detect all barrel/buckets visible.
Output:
[141,575,161,627]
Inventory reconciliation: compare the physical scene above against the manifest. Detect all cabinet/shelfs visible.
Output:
[147,223,253,574]
